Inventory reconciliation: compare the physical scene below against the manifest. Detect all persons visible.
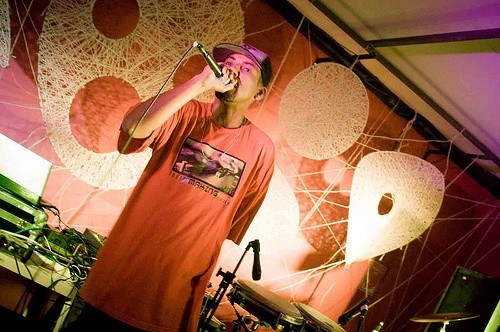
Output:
[62,41,276,332]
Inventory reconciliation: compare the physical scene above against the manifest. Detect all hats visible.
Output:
[213,43,272,86]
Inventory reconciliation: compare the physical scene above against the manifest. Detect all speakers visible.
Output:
[424,265,500,332]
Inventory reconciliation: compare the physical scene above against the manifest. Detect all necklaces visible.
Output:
[200,116,244,162]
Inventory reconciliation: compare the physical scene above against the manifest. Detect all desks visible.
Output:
[0,247,77,332]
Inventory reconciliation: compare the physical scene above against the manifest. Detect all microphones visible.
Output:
[196,43,231,85]
[252,239,261,281]
[338,298,370,325]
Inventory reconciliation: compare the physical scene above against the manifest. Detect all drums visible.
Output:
[229,279,304,331]
[290,302,346,332]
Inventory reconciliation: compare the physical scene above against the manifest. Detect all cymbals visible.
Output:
[410,312,481,322]
